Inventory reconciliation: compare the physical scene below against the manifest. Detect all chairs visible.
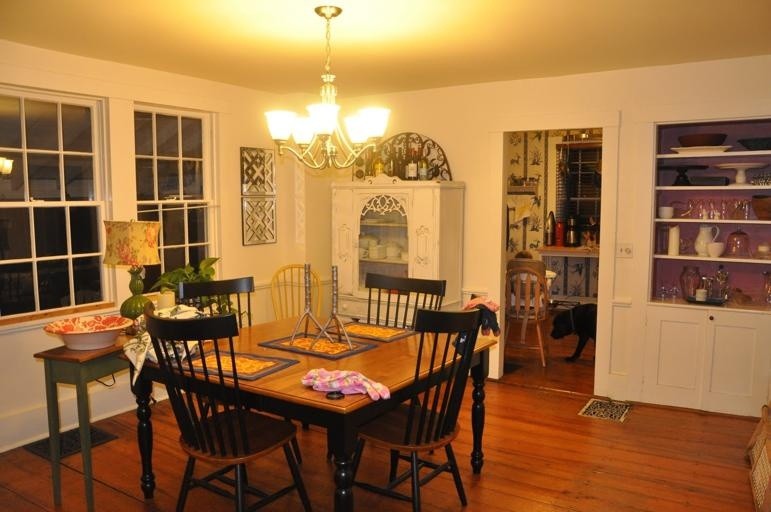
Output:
[314,272,447,462]
[144,313,314,511]
[270,264,322,317]
[351,308,482,512]
[504,266,550,369]
[507,259,549,350]
[174,276,256,423]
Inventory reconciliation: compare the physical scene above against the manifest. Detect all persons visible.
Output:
[508,251,543,310]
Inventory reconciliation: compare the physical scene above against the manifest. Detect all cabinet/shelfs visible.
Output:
[642,118,771,419]
[329,181,465,329]
[535,128,603,305]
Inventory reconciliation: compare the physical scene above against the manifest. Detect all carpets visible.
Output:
[23,422,118,460]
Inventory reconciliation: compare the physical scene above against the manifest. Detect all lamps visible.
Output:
[0,157,15,177]
[264,5,391,170]
[102,219,162,335]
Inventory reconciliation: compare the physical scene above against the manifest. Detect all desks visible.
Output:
[32,334,140,512]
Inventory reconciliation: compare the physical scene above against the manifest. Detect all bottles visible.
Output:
[363,144,430,181]
[678,264,730,297]
[761,271,771,306]
[519,177,538,187]
[543,212,580,247]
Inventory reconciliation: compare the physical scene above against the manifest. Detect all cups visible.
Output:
[695,288,708,301]
[706,242,725,258]
[687,198,753,220]
[658,206,674,219]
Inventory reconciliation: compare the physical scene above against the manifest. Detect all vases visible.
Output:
[680,264,703,300]
[578,398,632,422]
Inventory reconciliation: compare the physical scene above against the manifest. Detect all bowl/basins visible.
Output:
[737,135,771,150]
[42,314,136,351]
[677,133,728,147]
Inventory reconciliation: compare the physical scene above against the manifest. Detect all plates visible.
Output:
[358,234,409,262]
[361,217,385,224]
[683,295,727,306]
[668,144,733,153]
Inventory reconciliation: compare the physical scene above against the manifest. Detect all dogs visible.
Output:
[550,303,598,363]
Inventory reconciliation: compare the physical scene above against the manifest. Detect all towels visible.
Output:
[303,367,390,401]
